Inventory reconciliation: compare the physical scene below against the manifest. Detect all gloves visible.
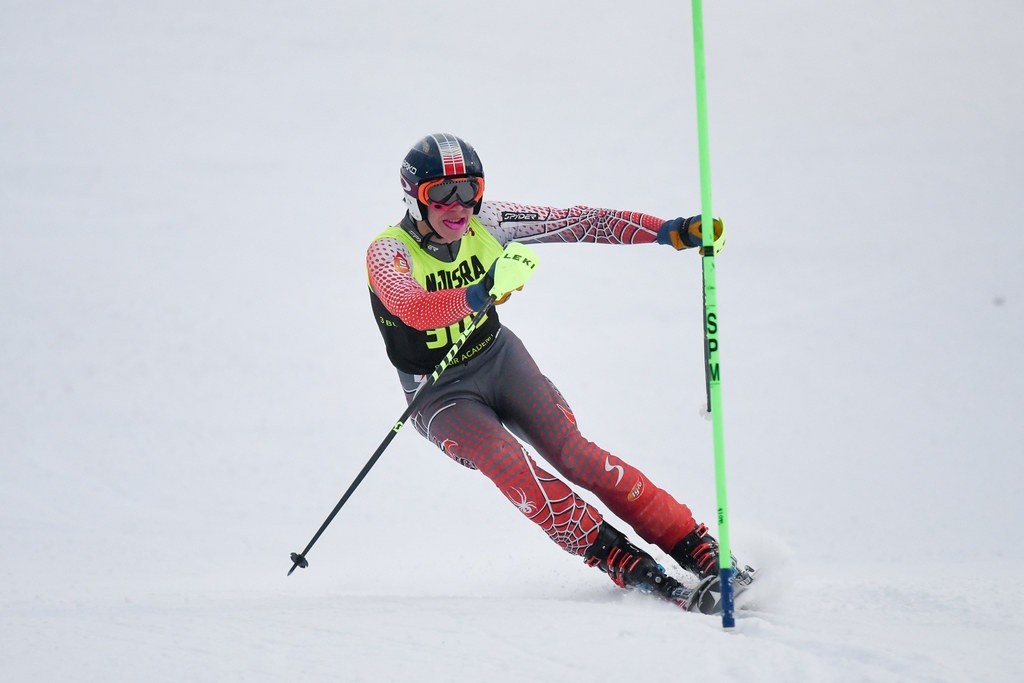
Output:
[466,257,524,312]
[657,213,722,255]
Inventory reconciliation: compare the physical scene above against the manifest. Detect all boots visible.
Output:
[669,523,738,583]
[583,519,696,611]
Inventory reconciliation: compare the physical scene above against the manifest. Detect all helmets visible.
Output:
[400,133,486,222]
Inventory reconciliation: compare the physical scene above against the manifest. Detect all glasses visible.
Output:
[417,176,484,210]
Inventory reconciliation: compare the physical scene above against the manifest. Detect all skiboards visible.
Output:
[684,575,717,611]
[698,573,750,616]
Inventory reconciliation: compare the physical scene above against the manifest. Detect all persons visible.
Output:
[366,133,756,618]
